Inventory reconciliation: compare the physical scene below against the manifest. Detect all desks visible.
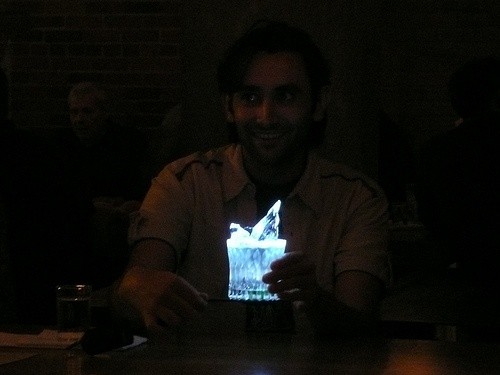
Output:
[0,216,500,375]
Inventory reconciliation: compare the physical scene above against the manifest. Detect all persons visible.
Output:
[109,20,393,343]
[0,68,147,292]
[415,58,500,337]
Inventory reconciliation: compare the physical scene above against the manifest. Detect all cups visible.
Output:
[226,239,287,300]
[56,283,93,333]
